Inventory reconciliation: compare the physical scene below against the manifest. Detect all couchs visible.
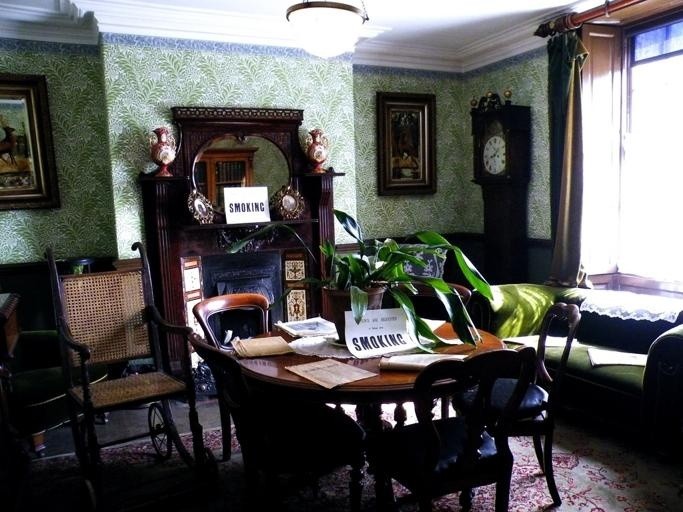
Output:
[472,280,683,464]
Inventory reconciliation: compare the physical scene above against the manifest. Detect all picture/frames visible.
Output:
[0,71,59,213]
[272,183,306,220]
[374,89,440,198]
[187,189,213,226]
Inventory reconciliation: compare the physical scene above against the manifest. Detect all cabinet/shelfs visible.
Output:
[194,146,261,218]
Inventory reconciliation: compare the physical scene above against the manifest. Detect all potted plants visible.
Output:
[224,206,496,352]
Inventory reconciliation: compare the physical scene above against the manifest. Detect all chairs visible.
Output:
[375,350,541,512]
[190,294,368,512]
[359,272,471,484]
[462,294,587,512]
[45,241,213,512]
[0,253,109,455]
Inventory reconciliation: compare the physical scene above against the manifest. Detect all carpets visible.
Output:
[2,405,680,510]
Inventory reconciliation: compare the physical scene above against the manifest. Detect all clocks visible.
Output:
[468,86,534,286]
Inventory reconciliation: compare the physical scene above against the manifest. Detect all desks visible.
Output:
[227,317,506,510]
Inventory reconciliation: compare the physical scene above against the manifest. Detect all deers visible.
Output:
[0,126,19,166]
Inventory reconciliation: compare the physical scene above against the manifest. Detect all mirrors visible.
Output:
[191,132,291,218]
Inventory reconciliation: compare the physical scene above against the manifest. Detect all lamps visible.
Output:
[284,0,371,62]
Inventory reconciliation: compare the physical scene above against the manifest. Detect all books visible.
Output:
[378,354,468,372]
